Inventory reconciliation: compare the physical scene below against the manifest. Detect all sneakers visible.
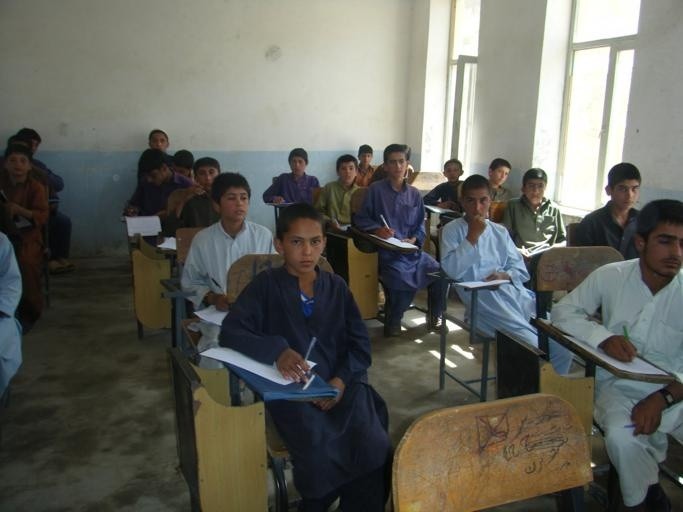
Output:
[428,317,449,335]
[385,322,404,336]
[51,259,73,275]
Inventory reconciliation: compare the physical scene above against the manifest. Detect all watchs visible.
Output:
[660,386,675,408]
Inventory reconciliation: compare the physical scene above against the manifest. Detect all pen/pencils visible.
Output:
[619,424,636,428]
[211,278,226,296]
[380,214,390,230]
[302,373,318,390]
[623,325,629,339]
[305,336,317,362]
[0,190,9,201]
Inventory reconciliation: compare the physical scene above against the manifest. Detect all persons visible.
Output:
[218,204,390,512]
[126,128,219,246]
[258,148,320,214]
[573,161,642,264]
[549,198,682,512]
[500,167,566,316]
[179,173,275,313]
[422,157,511,212]
[319,141,449,337]
[440,175,573,378]
[0,127,72,404]
